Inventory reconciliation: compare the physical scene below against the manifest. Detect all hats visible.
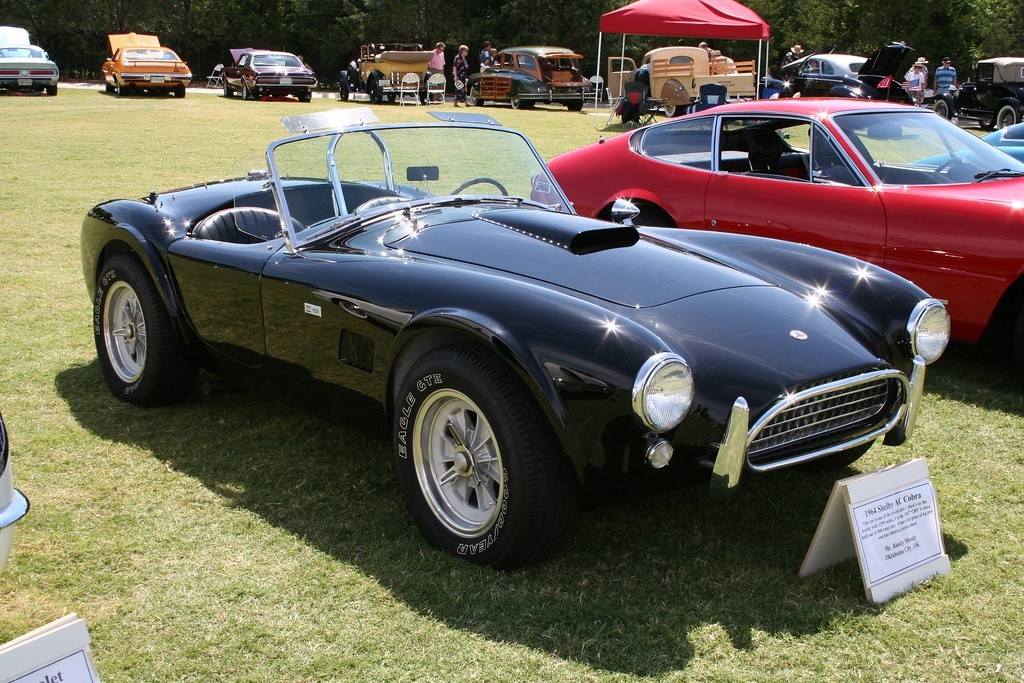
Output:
[912,62,923,66]
[915,57,929,63]
[712,50,721,56]
[791,45,804,54]
[942,57,951,62]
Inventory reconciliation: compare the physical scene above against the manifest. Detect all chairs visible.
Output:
[425,73,447,106]
[606,82,664,126]
[585,75,604,103]
[399,72,421,106]
[807,125,877,185]
[739,128,790,178]
[355,194,408,216]
[686,83,734,130]
[194,205,302,246]
[206,64,224,89]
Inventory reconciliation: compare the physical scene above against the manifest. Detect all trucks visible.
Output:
[608,45,757,118]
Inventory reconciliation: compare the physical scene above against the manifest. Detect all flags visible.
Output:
[901,78,919,89]
[877,75,892,90]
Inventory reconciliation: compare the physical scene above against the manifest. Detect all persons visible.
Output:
[453,45,471,107]
[298,55,312,70]
[428,42,446,104]
[480,41,497,73]
[782,45,804,75]
[904,57,957,103]
[634,63,650,85]
[698,42,738,74]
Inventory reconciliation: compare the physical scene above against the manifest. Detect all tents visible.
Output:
[594,0,770,129]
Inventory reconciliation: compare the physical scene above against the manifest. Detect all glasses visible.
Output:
[466,50,469,52]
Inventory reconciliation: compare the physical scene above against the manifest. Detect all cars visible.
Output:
[101,31,193,103]
[0,25,62,97]
[528,94,1024,387]
[932,56,1024,130]
[466,45,595,111]
[78,104,953,571]
[758,41,918,103]
[221,47,319,103]
[337,42,436,104]
